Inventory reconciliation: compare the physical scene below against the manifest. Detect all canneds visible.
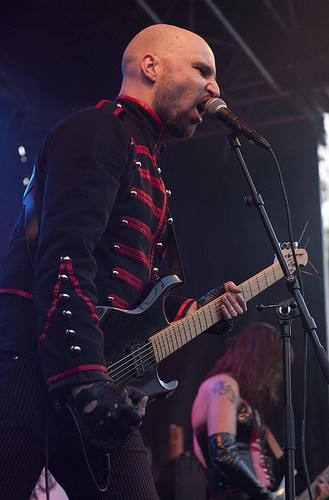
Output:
[169,423,184,459]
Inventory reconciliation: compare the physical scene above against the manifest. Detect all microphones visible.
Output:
[205,98,271,150]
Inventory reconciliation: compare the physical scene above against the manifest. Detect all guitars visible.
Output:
[267,465,329,500]
[52,242,310,458]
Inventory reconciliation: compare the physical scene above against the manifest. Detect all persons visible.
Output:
[0,23,247,500]
[190,322,328,500]
